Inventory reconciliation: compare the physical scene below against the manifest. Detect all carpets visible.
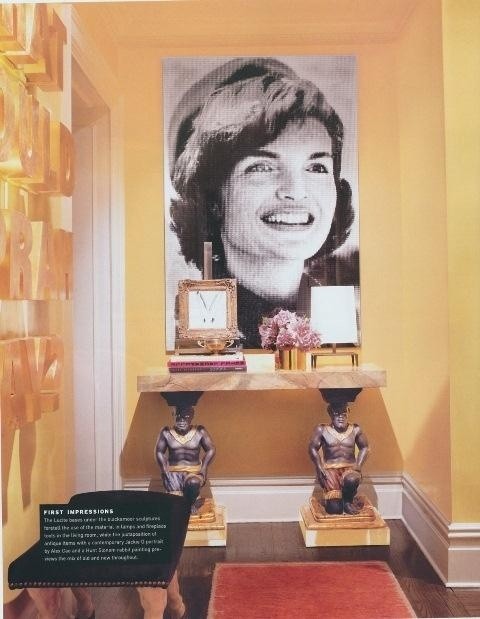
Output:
[206,561,421,618]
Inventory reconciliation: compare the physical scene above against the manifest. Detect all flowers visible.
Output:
[258,309,322,351]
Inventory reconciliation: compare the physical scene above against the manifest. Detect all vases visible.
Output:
[280,347,307,371]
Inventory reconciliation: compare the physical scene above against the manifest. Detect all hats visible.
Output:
[167,57,300,186]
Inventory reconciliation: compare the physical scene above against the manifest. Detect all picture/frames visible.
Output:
[178,278,238,339]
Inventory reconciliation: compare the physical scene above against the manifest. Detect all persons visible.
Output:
[167,57,359,349]
[154,405,216,514]
[308,401,369,515]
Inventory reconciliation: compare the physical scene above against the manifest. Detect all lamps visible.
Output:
[310,285,359,370]
